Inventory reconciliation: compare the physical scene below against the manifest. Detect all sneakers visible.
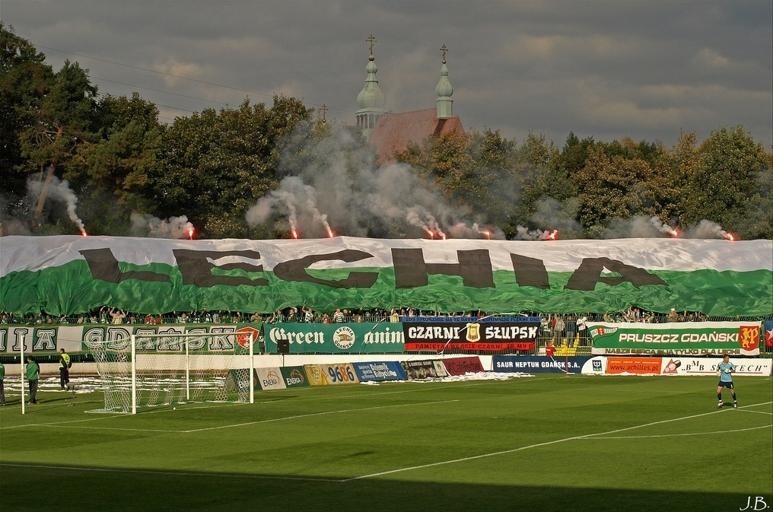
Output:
[718,400,723,406]
[734,400,737,408]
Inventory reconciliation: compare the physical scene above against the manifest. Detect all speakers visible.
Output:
[278,340,289,352]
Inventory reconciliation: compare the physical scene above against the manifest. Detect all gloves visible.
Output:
[725,369,731,374]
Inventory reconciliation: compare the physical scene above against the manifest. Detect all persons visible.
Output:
[540,307,705,348]
[59,348,72,390]
[0,363,6,406]
[0,297,485,324]
[716,354,737,408]
[546,341,557,357]
[26,356,40,403]
